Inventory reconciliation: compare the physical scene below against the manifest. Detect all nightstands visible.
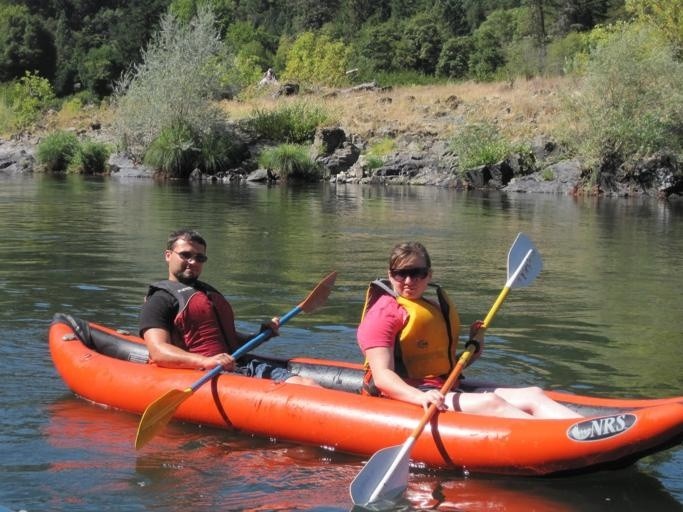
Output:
[350,233,542,504]
[135,270,335,450]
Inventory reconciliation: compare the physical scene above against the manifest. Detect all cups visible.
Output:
[169,248,207,262]
[389,267,429,281]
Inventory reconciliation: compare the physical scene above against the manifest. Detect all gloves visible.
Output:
[48,313,683,476]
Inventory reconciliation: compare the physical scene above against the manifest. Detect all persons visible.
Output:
[355,241,588,419]
[139,229,326,389]
